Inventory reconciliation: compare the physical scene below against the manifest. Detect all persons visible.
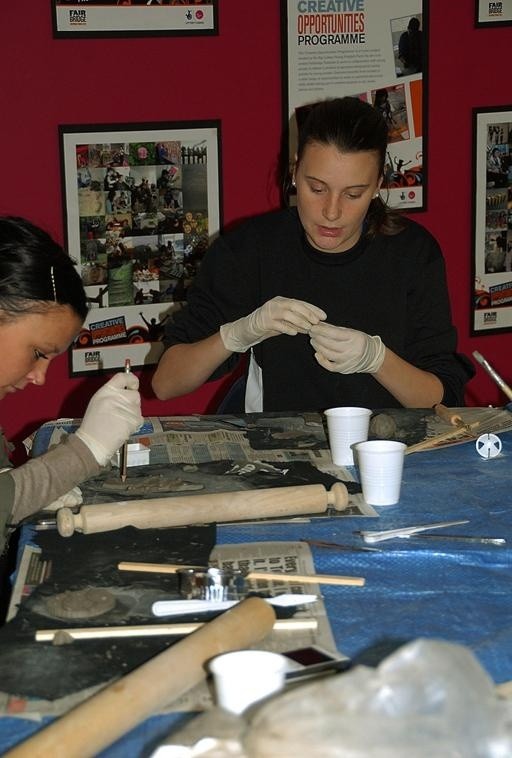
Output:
[373,88,397,125]
[148,93,476,412]
[1,211,147,550]
[393,156,412,172]
[485,121,511,277]
[79,135,206,345]
[397,16,424,72]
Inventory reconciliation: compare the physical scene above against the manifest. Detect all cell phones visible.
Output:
[280,645,353,685]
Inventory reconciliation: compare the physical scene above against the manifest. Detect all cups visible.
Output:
[352,440,405,505]
[324,407,371,466]
[179,568,240,601]
[207,649,290,715]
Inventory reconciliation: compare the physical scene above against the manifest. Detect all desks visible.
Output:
[0,405,511,757]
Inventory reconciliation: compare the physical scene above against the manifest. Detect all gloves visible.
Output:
[74,373,146,467]
[308,321,386,375]
[219,296,328,353]
[1,467,84,512]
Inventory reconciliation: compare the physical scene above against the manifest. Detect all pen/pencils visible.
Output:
[304,540,382,552]
[120,359,130,483]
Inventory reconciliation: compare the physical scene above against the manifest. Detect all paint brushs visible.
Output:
[473,350,512,400]
